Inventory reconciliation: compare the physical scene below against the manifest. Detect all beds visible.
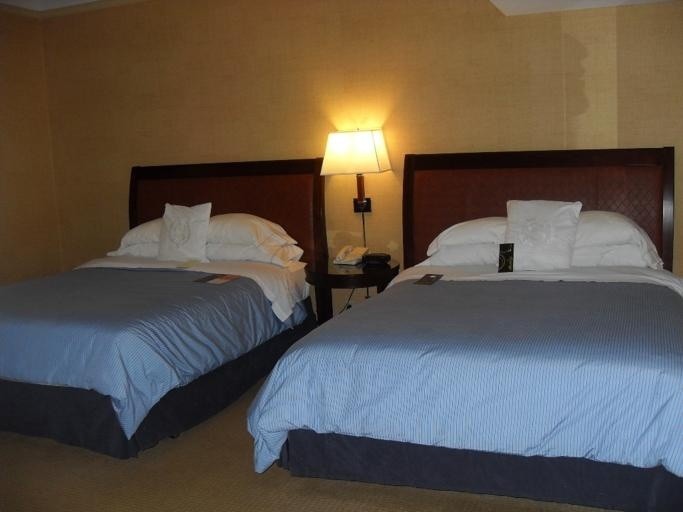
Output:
[1,155,333,458]
[247,148,683,512]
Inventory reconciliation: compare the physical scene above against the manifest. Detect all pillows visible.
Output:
[426,198,665,271]
[105,200,304,270]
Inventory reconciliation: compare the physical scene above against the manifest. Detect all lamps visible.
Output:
[318,127,393,213]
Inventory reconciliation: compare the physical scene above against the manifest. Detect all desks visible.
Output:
[304,258,400,326]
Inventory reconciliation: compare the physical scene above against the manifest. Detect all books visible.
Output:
[192,273,240,285]
[413,272,443,284]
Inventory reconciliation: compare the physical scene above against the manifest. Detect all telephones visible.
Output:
[333,245,369,265]
